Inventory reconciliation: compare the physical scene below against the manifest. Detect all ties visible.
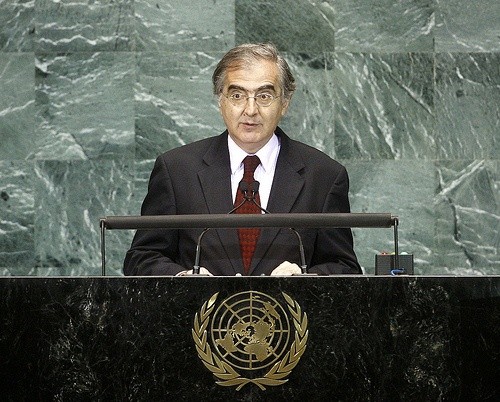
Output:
[233,154,262,277]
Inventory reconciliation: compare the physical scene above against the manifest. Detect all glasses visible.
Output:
[221,92,286,107]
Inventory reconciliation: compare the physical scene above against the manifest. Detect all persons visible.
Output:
[121,40,364,276]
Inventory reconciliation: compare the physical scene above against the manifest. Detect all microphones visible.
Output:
[251,182,306,266]
[194,180,249,266]
